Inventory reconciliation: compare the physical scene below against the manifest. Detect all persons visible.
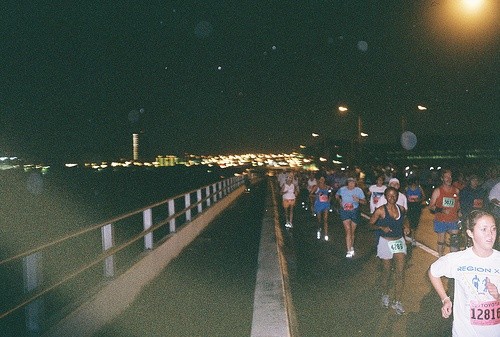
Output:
[334,178,366,259]
[426,167,462,267]
[427,210,500,337]
[368,186,412,318]
[271,166,500,260]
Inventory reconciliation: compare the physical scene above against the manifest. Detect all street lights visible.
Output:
[339,107,363,168]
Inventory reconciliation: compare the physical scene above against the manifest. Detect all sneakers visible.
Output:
[382,294,390,308]
[392,300,405,315]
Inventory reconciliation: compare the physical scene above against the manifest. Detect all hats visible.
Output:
[389,178,399,184]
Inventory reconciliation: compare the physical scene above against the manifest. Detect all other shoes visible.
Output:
[324,236,329,242]
[285,224,291,228]
[317,231,321,240]
[290,224,293,228]
[346,250,354,258]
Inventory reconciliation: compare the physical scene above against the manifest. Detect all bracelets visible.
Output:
[442,296,451,304]
